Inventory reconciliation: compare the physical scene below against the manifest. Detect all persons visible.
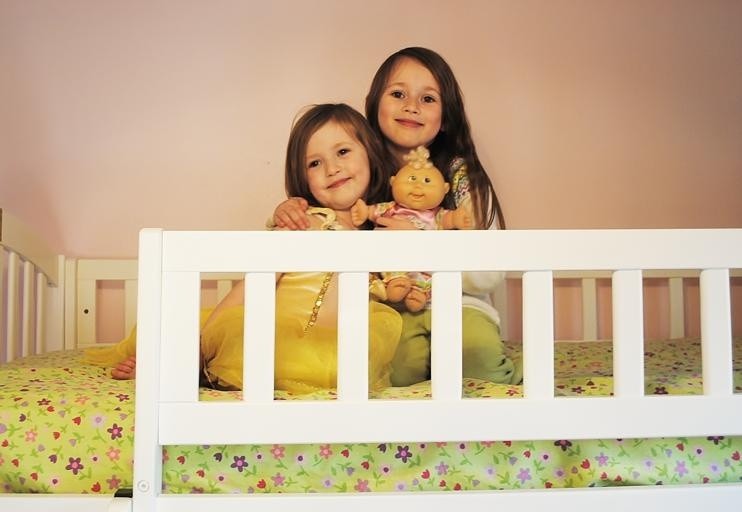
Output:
[349,146,474,313]
[78,102,405,395]
[262,46,523,388]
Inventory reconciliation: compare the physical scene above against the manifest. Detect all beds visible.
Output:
[1,212,741,494]
